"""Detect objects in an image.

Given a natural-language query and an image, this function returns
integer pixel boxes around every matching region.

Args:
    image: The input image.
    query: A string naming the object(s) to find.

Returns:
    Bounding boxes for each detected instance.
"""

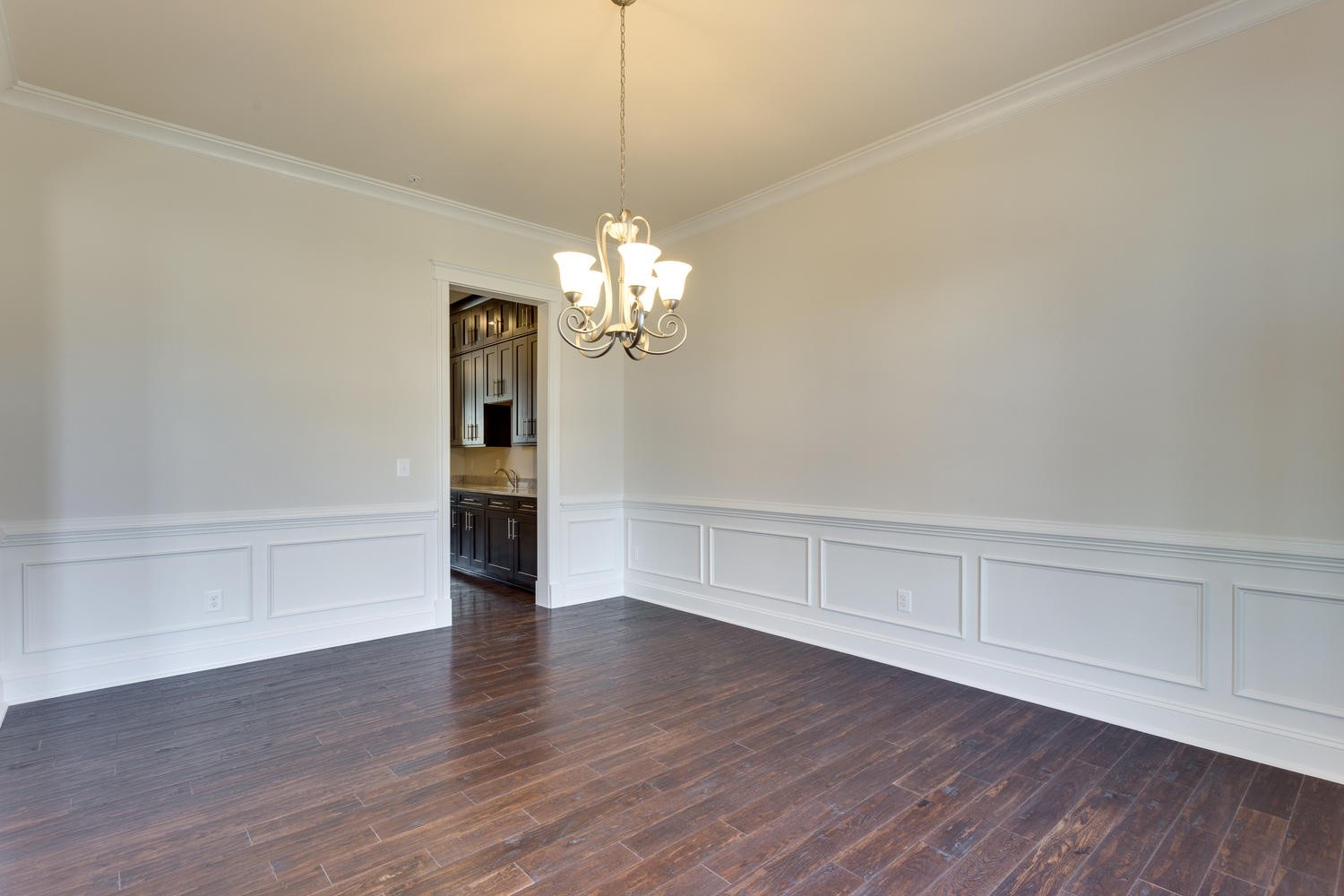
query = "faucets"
[494,468,518,488]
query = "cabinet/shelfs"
[451,491,537,595]
[449,294,538,449]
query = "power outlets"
[495,459,500,469]
[469,459,474,469]
[896,589,911,614]
[206,590,222,614]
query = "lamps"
[552,0,690,361]
